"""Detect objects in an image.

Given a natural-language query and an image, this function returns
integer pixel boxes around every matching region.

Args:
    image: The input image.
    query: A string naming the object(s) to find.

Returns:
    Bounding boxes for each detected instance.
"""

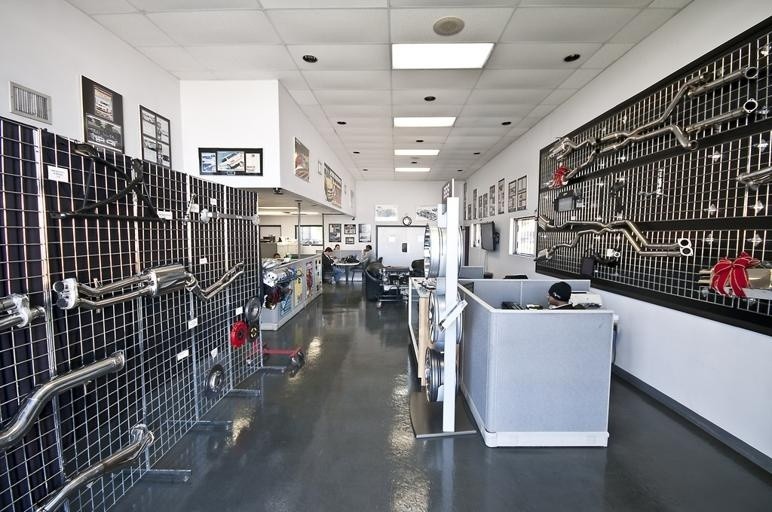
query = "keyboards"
[512,303,527,310]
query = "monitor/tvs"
[480,223,495,251]
[463,282,474,293]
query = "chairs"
[365,269,383,302]
[378,257,383,264]
[410,259,424,277]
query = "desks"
[385,267,409,285]
[384,284,409,302]
[334,262,361,285]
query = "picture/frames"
[329,224,341,242]
[359,224,371,242]
[345,237,354,244]
[344,224,356,234]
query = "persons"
[322,247,345,283]
[333,244,340,250]
[358,245,376,268]
[547,281,574,310]
[274,253,280,259]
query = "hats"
[548,282,571,302]
